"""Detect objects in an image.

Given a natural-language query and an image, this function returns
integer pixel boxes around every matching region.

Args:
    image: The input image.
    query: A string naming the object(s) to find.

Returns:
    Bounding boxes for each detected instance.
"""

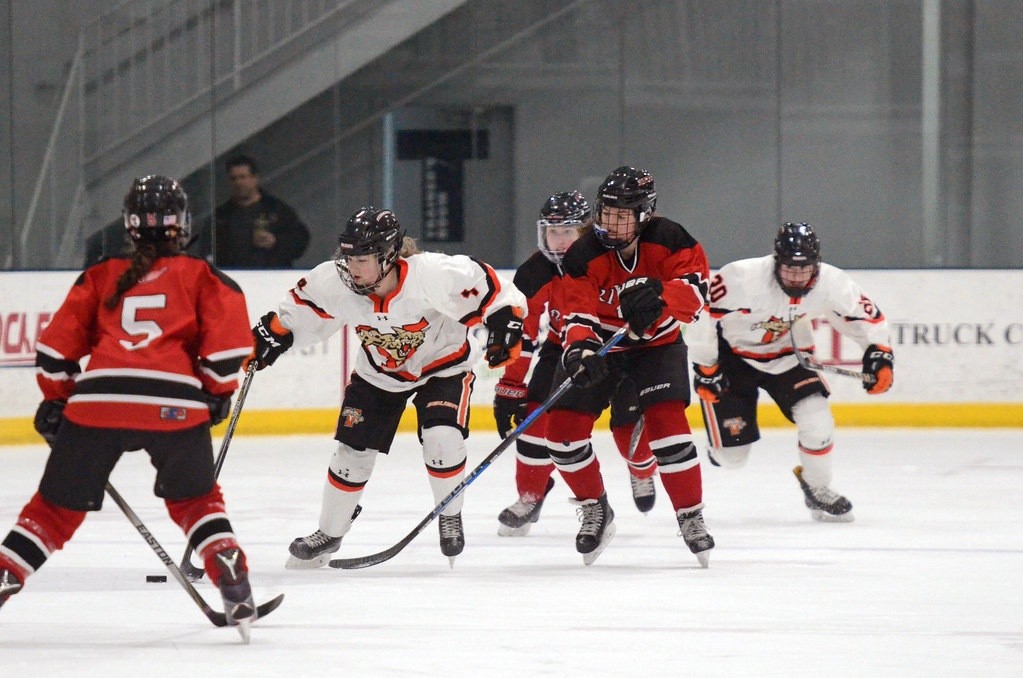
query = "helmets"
[591,166,657,251]
[333,207,403,295]
[123,175,189,248]
[537,189,589,262]
[774,222,821,297]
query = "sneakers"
[676,502,714,567]
[630,473,656,516]
[439,511,465,569]
[569,473,616,565]
[793,465,854,522]
[215,548,258,644]
[284,505,362,569]
[497,477,555,535]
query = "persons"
[681,221,895,522]
[545,165,716,569]
[494,190,658,537]
[200,156,310,270]
[242,206,528,570]
[0,174,256,646]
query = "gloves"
[241,311,294,374]
[861,344,895,394]
[203,388,230,426]
[483,306,525,368]
[617,277,665,338]
[34,399,67,447]
[692,361,729,402]
[563,340,610,387]
[492,378,529,440]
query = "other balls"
[146,574,168,583]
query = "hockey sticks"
[179,358,258,581]
[328,293,665,570]
[45,412,285,629]
[787,311,876,383]
[628,413,643,460]
